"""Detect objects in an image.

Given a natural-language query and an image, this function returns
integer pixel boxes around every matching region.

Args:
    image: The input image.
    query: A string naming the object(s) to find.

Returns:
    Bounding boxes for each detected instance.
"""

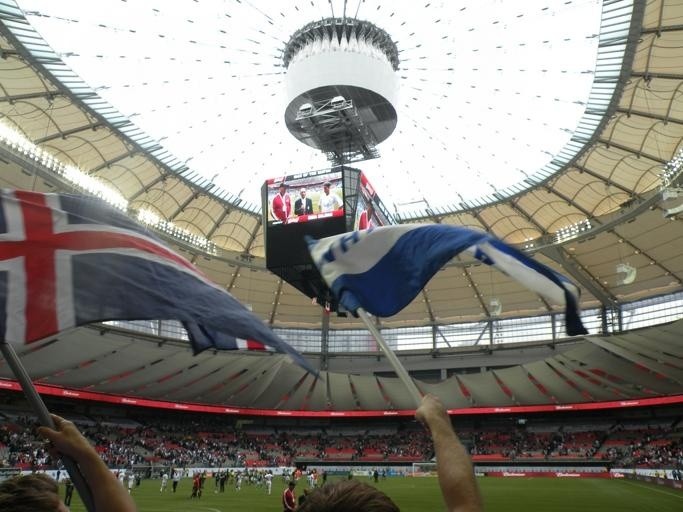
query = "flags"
[0,188,326,383]
[302,220,591,337]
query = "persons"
[1,390,681,512]
[294,188,313,215]
[317,182,344,213]
[269,182,292,224]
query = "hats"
[278,183,289,188]
[323,181,331,186]
